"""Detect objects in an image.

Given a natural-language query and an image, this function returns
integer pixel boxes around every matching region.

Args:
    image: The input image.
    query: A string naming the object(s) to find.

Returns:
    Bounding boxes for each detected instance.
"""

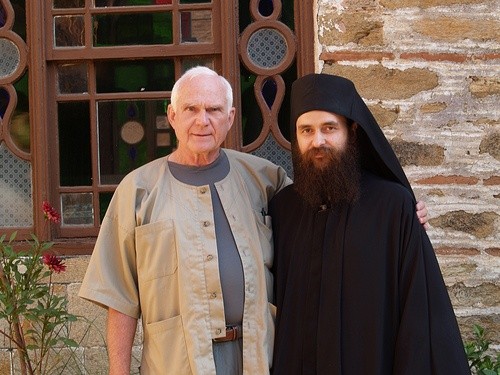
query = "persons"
[77,65,432,375]
[267,72,471,375]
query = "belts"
[211,324,242,342]
